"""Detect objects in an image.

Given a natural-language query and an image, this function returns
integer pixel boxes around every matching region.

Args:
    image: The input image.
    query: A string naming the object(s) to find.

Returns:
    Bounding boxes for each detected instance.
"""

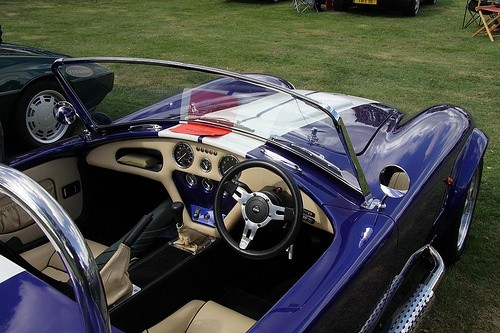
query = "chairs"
[471,0,500,43]
[462,0,499,32]
[140,300,258,332]
[0,231,135,313]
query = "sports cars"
[0,58,487,333]
[0,42,114,151]
